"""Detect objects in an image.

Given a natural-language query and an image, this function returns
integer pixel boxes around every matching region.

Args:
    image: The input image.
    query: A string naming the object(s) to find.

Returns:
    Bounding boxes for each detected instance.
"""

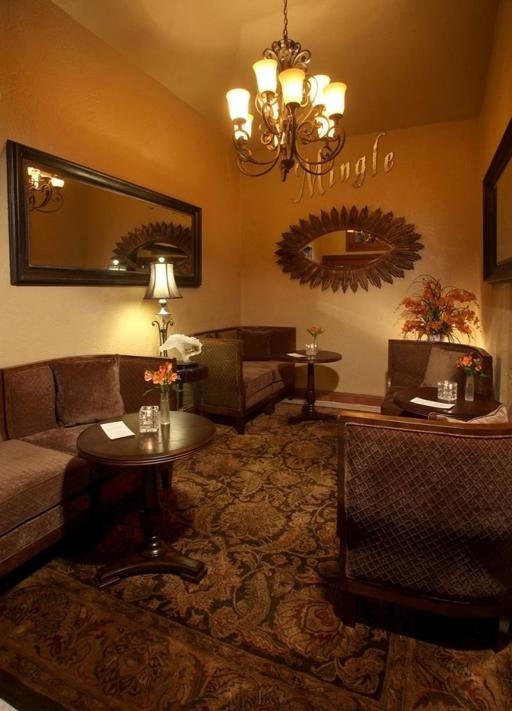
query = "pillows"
[238,328,274,361]
[428,404,509,426]
[49,354,125,427]
[418,346,468,389]
[3,365,58,440]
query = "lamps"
[142,257,183,357]
[225,0,349,183]
[27,166,65,214]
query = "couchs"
[336,407,511,626]
[185,326,297,434]
[1,354,178,578]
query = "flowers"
[307,325,325,340]
[455,352,488,378]
[141,361,184,397]
[395,273,484,343]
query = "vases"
[428,332,444,344]
[464,375,475,403]
[159,389,171,425]
[305,339,319,356]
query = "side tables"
[174,364,209,416]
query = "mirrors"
[6,138,203,289]
[107,220,194,275]
[273,204,425,294]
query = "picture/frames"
[482,116,512,284]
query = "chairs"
[381,334,493,416]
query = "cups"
[304,342,320,357]
[138,404,161,436]
[437,379,460,400]
